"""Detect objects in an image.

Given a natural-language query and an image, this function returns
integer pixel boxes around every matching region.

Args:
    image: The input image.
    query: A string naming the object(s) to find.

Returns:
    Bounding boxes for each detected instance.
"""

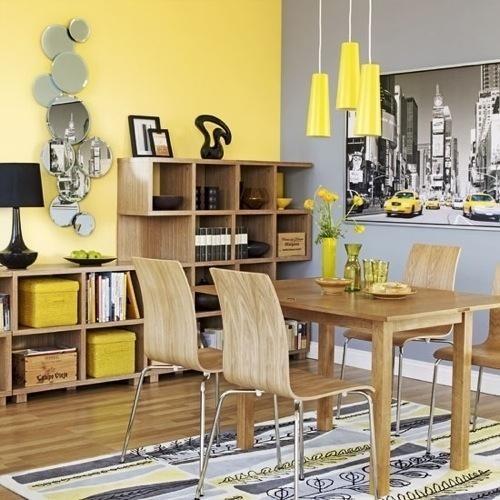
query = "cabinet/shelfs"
[0,259,148,407]
[117,155,313,383]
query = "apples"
[71,249,101,260]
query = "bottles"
[344,243,363,292]
[240,187,269,209]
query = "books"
[12,346,76,357]
[0,293,10,331]
[195,225,250,262]
[87,273,141,324]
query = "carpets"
[0,396,500,500]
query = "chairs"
[193,268,379,500]
[119,256,283,497]
[336,244,462,437]
[426,263,500,455]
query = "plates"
[63,256,118,266]
[314,276,353,288]
[365,289,417,300]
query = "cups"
[371,259,391,286]
[362,259,376,284]
[195,186,202,211]
[204,185,219,210]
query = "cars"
[346,190,370,210]
[422,195,465,210]
[383,190,423,218]
[462,193,500,222]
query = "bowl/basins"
[276,197,293,210]
[152,195,183,210]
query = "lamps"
[0,163,45,269]
[305,0,382,138]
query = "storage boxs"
[19,278,80,328]
[277,232,305,256]
[14,352,77,387]
[86,329,137,378]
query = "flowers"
[304,186,366,245]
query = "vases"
[322,238,336,278]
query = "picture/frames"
[128,115,160,157]
[148,129,173,157]
[344,59,500,233]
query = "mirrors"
[33,18,113,236]
